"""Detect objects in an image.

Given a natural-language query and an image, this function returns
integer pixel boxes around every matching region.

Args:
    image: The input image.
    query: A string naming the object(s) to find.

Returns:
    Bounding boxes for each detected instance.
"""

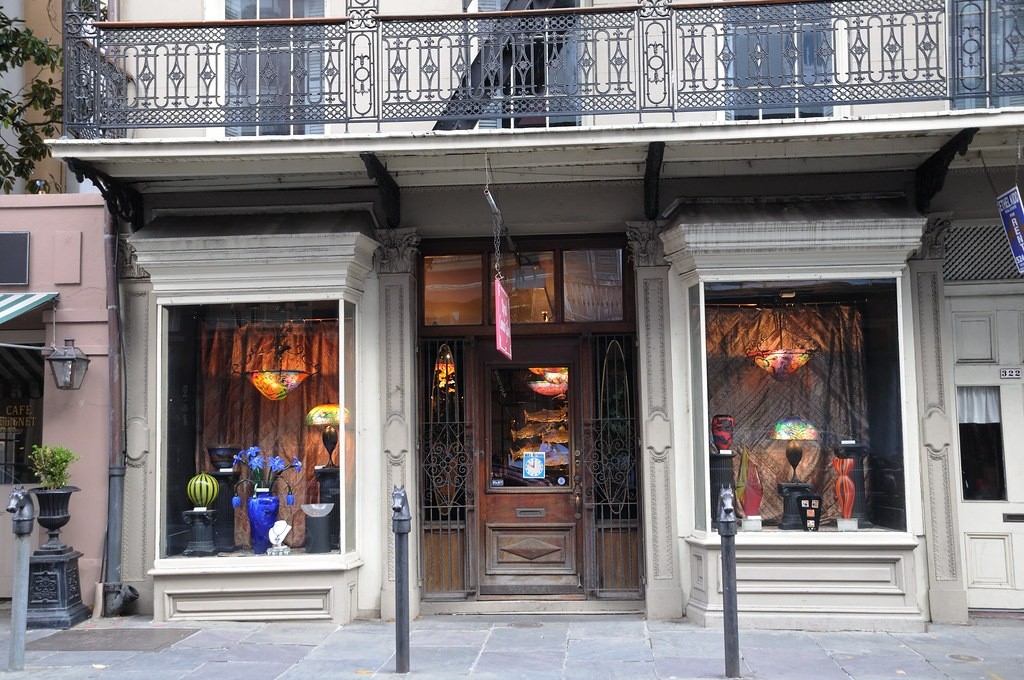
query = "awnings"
[0,292,57,326]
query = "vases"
[247,488,280,554]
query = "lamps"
[527,367,568,400]
[745,305,817,382]
[770,413,820,482]
[303,403,351,468]
[231,320,321,401]
[46,339,90,390]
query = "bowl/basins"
[300,503,335,517]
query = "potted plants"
[27,444,82,548]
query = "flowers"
[231,446,302,509]
